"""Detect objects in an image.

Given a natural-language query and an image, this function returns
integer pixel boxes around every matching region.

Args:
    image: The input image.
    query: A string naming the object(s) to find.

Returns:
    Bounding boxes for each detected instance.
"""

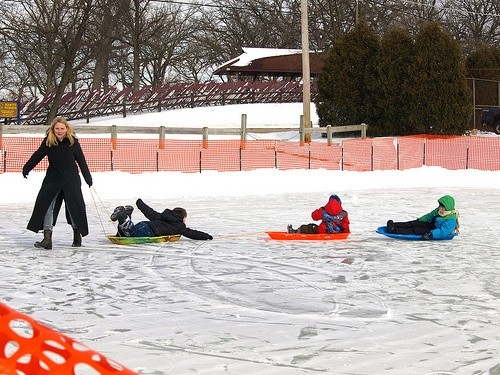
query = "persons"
[386,194,460,240]
[287,194,351,234]
[23,117,93,249]
[110,198,213,240]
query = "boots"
[72,227,82,246]
[35,231,53,249]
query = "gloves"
[208,235,213,240]
[421,233,432,241]
[23,170,29,179]
[86,181,93,187]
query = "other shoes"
[287,224,295,233]
[111,206,126,221]
[387,219,395,233]
[122,206,134,217]
